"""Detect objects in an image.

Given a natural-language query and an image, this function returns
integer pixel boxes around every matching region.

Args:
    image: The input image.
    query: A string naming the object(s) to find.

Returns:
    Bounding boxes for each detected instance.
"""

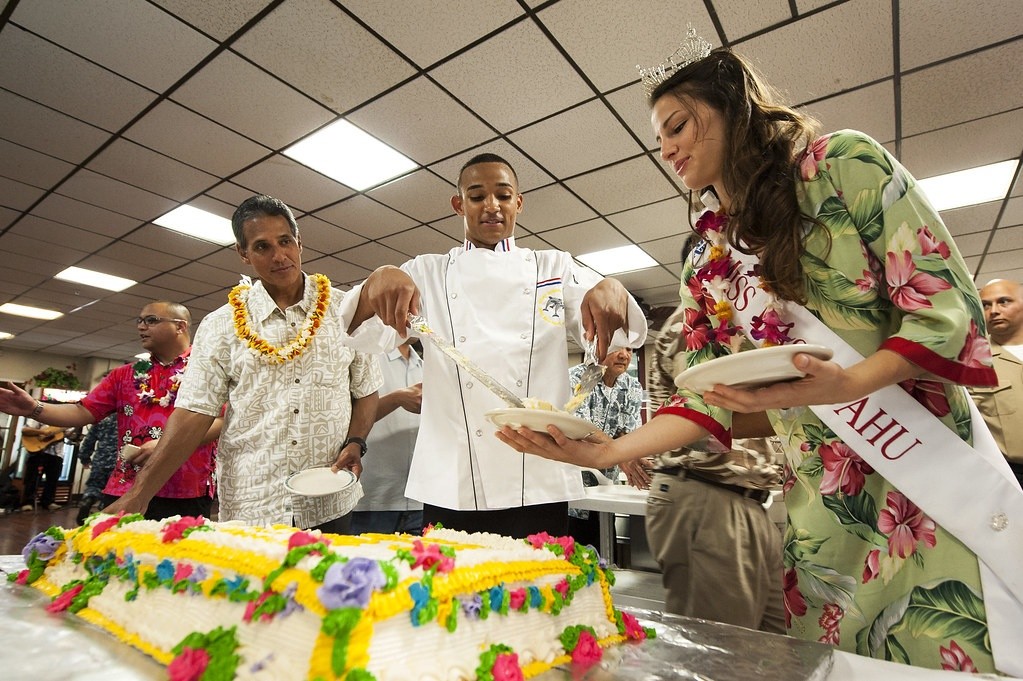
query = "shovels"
[567,325,608,414]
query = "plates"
[285,468,357,497]
[674,344,833,396]
[483,407,596,441]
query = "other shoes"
[22,505,32,510]
[47,503,62,510]
[76,499,92,525]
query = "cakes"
[520,398,555,413]
[8,511,657,681]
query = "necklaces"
[228,273,331,368]
[131,357,190,407]
[694,208,796,347]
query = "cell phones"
[121,444,141,461]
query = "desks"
[568,482,787,566]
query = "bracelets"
[341,437,368,458]
[31,400,44,420]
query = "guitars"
[22,426,77,453]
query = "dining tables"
[0,555,1000,681]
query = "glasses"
[137,315,187,324]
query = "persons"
[0,300,225,525]
[338,152,648,544]
[102,195,386,534]
[349,314,424,536]
[490,23,1022,681]
[568,317,652,517]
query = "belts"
[653,465,770,503]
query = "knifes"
[406,313,525,408]
[560,327,607,413]
